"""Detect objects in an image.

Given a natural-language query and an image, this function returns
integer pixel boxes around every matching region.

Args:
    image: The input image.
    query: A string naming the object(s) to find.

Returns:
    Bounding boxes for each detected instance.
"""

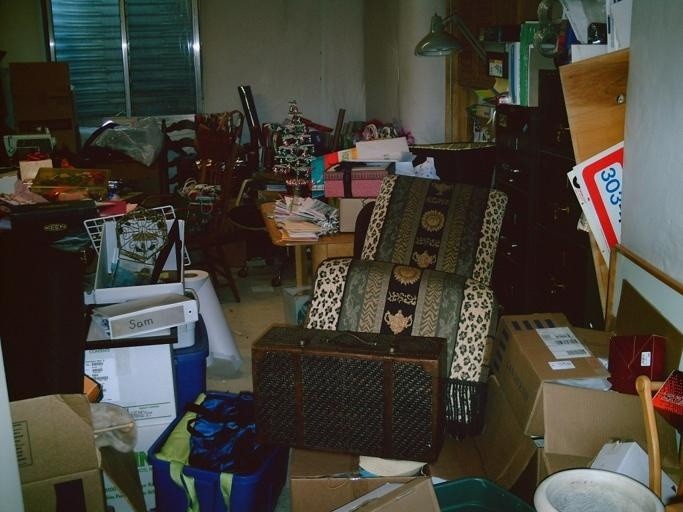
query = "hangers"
[415,16,465,57]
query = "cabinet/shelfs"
[526,1,625,330]
[0,199,99,400]
[447,0,530,316]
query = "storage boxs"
[10,394,104,512]
[173,315,209,413]
[87,218,186,306]
[571,329,622,370]
[146,386,287,512]
[492,314,613,438]
[333,481,406,511]
[432,476,536,511]
[542,381,681,512]
[650,369,681,437]
[289,448,442,511]
[608,335,667,398]
[473,375,540,492]
[355,476,440,511]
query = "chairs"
[143,117,242,304]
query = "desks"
[265,196,354,296]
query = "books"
[506,21,555,107]
[268,195,337,242]
